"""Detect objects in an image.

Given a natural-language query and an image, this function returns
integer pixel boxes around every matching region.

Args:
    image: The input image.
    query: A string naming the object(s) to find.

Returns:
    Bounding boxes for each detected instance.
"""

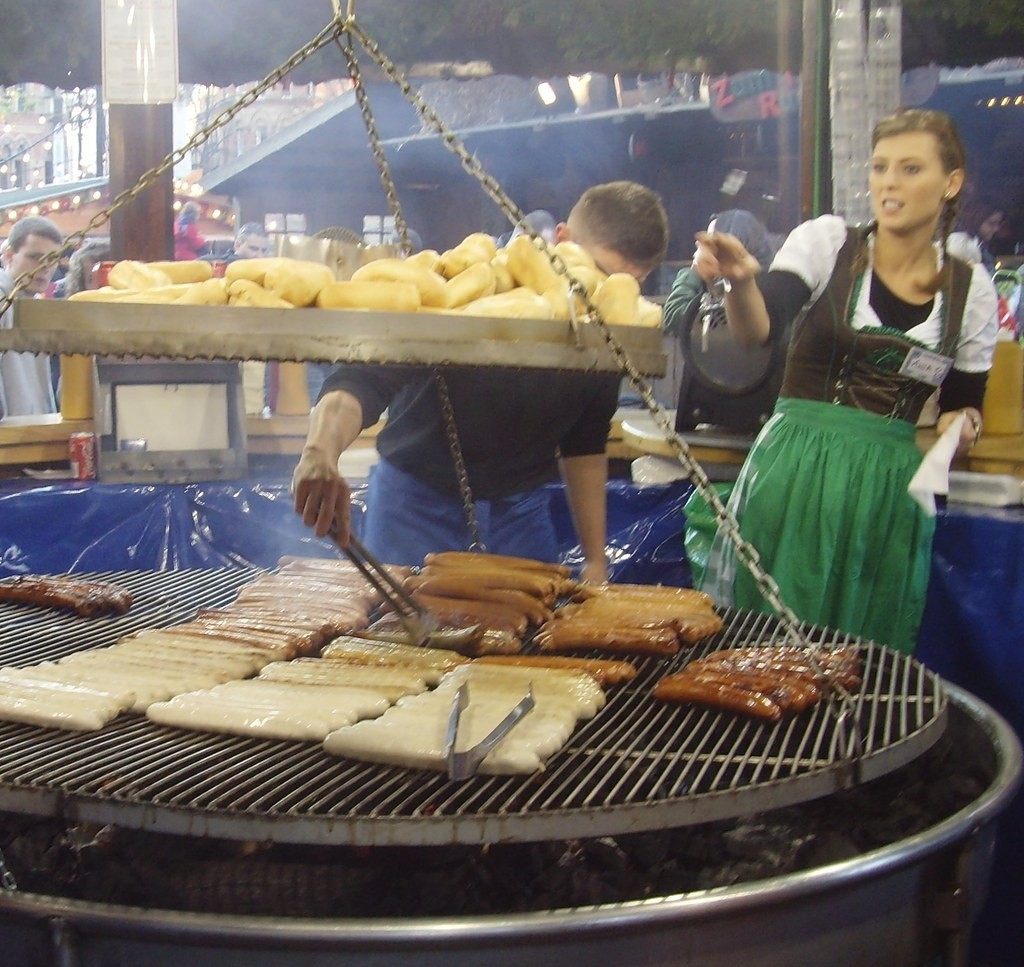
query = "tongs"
[445,677,535,781]
[288,482,440,645]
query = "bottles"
[980,327,1022,437]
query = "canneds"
[69,432,97,480]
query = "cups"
[569,72,710,115]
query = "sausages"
[0,552,861,777]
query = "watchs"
[957,408,981,453]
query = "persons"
[0,215,115,420]
[944,195,1005,297]
[194,220,268,264]
[696,109,1001,664]
[291,181,670,639]
[660,207,783,437]
[174,201,205,264]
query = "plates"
[23,464,71,479]
